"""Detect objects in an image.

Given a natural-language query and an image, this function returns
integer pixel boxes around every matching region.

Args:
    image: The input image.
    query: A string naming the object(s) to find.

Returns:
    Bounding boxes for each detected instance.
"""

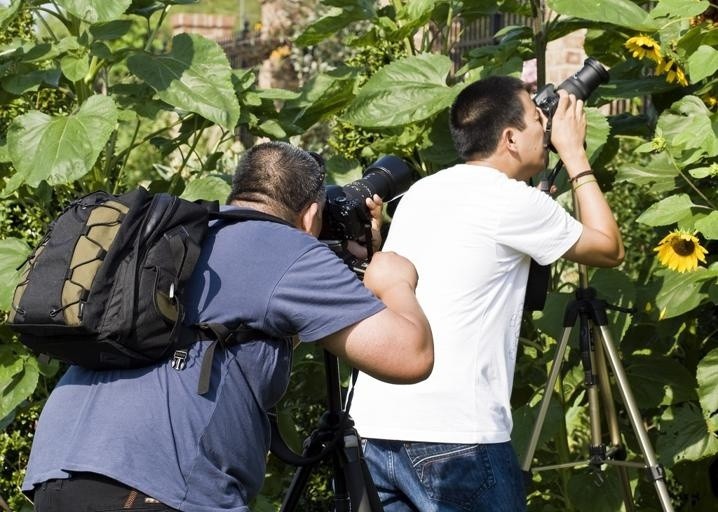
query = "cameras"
[532,56,609,151]
[322,154,411,243]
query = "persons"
[343,75,626,512]
[18,142,434,512]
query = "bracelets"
[567,169,595,184]
[572,179,599,192]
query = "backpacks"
[4,184,297,374]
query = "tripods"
[518,152,676,512]
[274,349,384,512]
